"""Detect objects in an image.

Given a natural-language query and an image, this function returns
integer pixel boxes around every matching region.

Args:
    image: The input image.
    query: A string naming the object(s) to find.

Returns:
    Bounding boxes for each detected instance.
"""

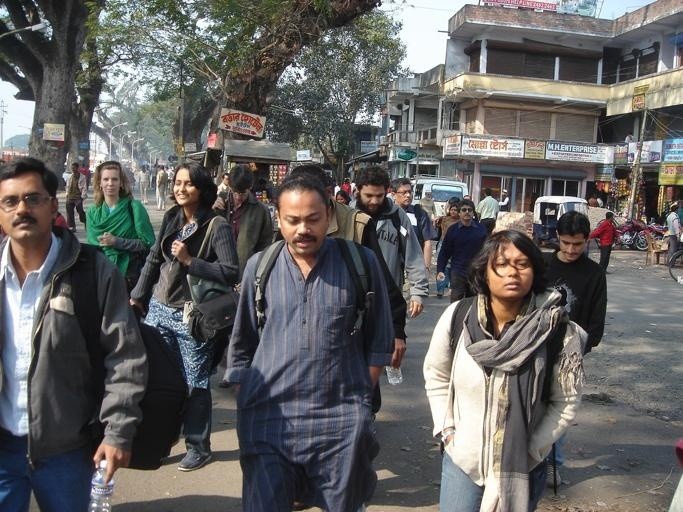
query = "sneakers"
[216,366,232,389]
[175,448,214,473]
[436,289,444,297]
[68,227,77,233]
[545,457,565,487]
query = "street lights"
[130,137,145,164]
[149,150,159,169]
[131,137,145,160]
[154,151,163,164]
[1,22,47,40]
[148,145,158,150]
[146,143,163,170]
[0,18,45,36]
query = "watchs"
[425,267,431,273]
[440,430,456,442]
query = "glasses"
[394,190,414,194]
[0,192,55,212]
[460,208,474,212]
[231,187,248,194]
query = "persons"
[84,160,157,291]
[0,157,146,512]
[463,195,479,222]
[447,197,461,205]
[255,178,274,201]
[498,189,511,212]
[223,176,397,512]
[130,162,238,471]
[213,165,279,310]
[290,165,411,412]
[678,201,683,228]
[424,230,584,511]
[669,196,682,209]
[351,165,430,317]
[333,175,357,208]
[667,203,683,267]
[65,163,92,231]
[432,206,460,296]
[418,190,438,221]
[437,199,488,306]
[126,164,175,213]
[535,210,608,486]
[217,173,231,192]
[475,188,499,235]
[588,212,615,274]
[388,177,432,313]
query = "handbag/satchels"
[123,198,151,294]
[189,218,240,347]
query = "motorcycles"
[594,212,652,252]
[650,225,668,251]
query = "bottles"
[385,366,404,386]
[89,459,115,511]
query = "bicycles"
[668,248,683,283]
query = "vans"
[410,178,468,227]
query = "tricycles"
[532,195,590,256]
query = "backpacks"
[65,244,185,472]
